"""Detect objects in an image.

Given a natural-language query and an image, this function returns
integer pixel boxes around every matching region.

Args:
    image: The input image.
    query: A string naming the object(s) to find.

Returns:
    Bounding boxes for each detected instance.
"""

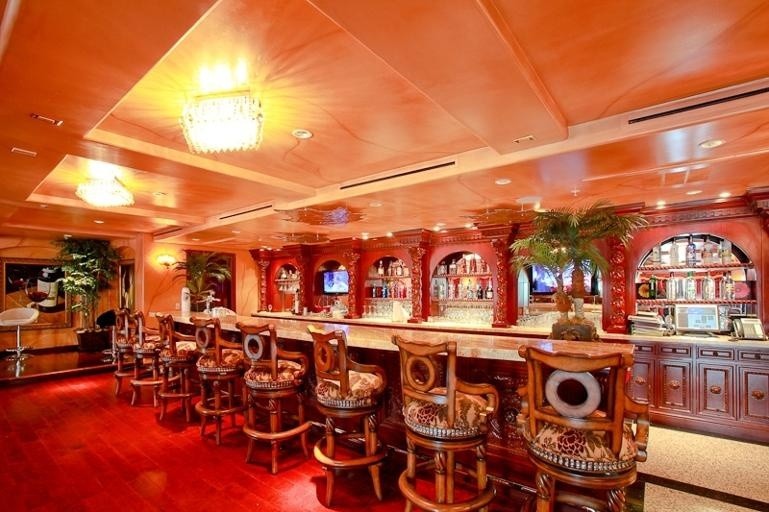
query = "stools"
[309,329,397,508]
[390,331,501,512]
[114,309,151,396]
[154,312,201,424]
[234,320,311,475]
[126,310,178,406]
[518,342,652,511]
[188,316,248,445]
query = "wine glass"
[7,269,51,323]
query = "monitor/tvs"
[321,269,349,295]
[530,258,592,293]
[675,303,720,337]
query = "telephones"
[732,318,766,341]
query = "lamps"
[76,91,262,210]
[157,254,177,268]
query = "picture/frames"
[0,257,74,331]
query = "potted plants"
[49,237,123,352]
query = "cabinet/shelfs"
[247,184,769,341]
[625,338,769,447]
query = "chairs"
[0,307,40,379]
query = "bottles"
[686,234,697,265]
[37,265,61,313]
[666,272,677,300]
[722,234,731,263]
[652,240,661,264]
[670,237,679,266]
[372,259,412,298]
[684,271,697,301]
[701,272,715,301]
[719,270,735,300]
[703,234,714,264]
[433,253,493,300]
[648,271,658,299]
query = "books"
[626,308,664,336]
